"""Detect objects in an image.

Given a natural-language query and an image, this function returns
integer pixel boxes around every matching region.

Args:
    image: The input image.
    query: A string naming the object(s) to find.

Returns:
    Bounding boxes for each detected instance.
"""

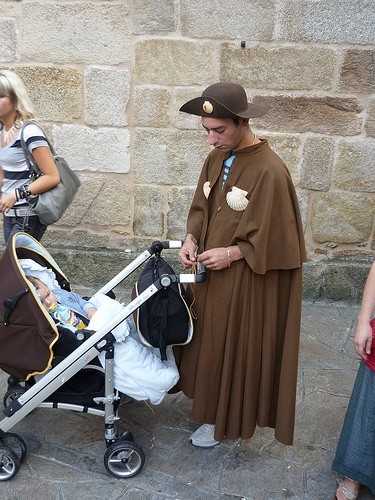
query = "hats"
[179,83,269,117]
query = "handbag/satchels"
[19,122,81,226]
[131,258,193,362]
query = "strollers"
[0,231,207,483]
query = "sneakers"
[189,422,221,447]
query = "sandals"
[335,476,359,500]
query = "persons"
[332,260,375,500]
[24,275,97,331]
[165,83,305,449]
[0,69,60,248]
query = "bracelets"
[15,184,31,201]
[227,246,230,267]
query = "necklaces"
[253,133,257,143]
